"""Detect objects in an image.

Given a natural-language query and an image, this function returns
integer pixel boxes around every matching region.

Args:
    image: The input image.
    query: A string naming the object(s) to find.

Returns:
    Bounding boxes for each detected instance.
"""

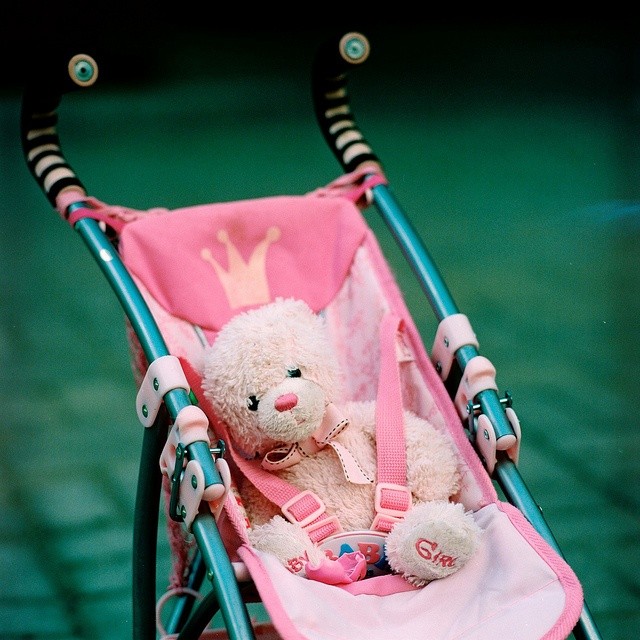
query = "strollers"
[21,27,604,640]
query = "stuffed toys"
[201,298,482,589]
